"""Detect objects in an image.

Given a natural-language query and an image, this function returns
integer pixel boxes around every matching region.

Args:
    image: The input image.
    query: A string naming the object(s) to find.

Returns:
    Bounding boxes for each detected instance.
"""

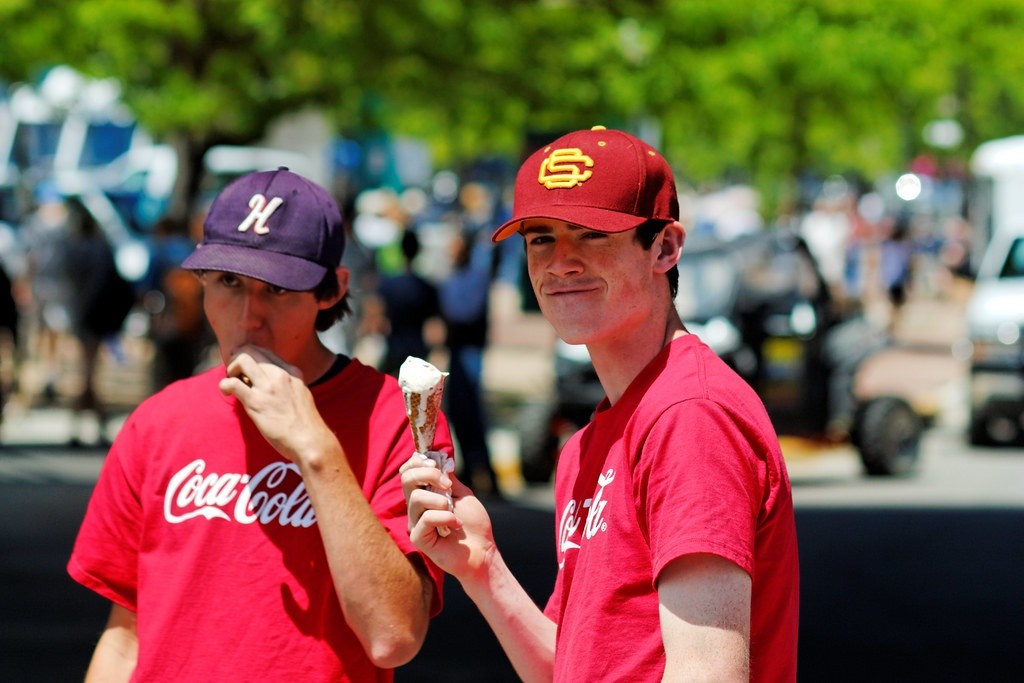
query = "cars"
[516,227,925,486]
[938,215,1024,448]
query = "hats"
[181,167,347,291]
[496,127,680,240]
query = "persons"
[0,195,492,482]
[400,124,799,683]
[66,166,454,683]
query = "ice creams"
[399,355,446,453]
